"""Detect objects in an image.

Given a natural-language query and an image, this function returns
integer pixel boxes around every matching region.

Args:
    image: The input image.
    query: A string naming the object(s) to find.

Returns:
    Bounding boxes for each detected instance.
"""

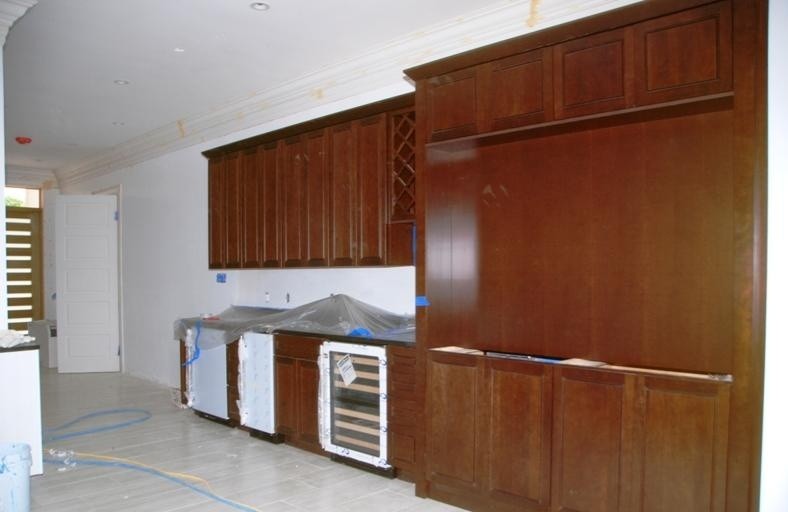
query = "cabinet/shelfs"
[424,45,554,141]
[179,318,417,484]
[555,1,734,121]
[426,346,555,511]
[207,112,388,269]
[552,358,733,510]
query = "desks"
[1,341,45,478]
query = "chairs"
[33,320,57,368]
